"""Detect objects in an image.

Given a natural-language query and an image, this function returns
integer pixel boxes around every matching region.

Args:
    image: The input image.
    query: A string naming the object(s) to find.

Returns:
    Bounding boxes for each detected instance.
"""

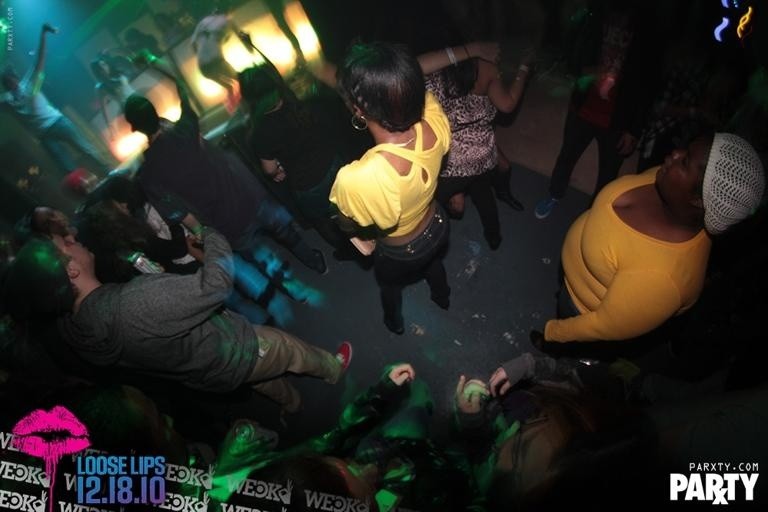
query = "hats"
[701,132,764,236]
[2,239,73,315]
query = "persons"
[318,32,456,332]
[2,340,767,512]
[5,220,352,408]
[0,2,768,321]
[526,128,766,364]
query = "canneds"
[234,422,279,449]
[131,255,164,275]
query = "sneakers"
[308,248,330,275]
[535,197,556,219]
[334,341,353,385]
[529,330,561,360]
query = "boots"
[496,169,523,210]
